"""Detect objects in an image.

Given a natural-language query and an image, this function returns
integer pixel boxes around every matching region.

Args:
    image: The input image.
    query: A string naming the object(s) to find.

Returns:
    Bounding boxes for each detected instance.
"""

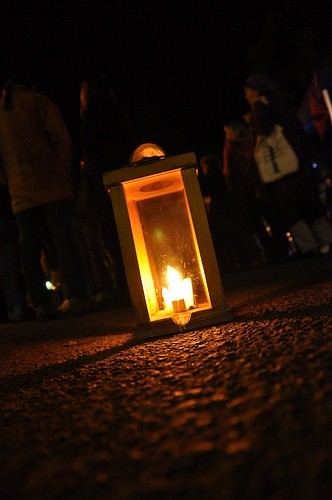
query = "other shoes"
[1,280,125,322]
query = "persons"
[1,58,100,321]
[223,72,332,259]
